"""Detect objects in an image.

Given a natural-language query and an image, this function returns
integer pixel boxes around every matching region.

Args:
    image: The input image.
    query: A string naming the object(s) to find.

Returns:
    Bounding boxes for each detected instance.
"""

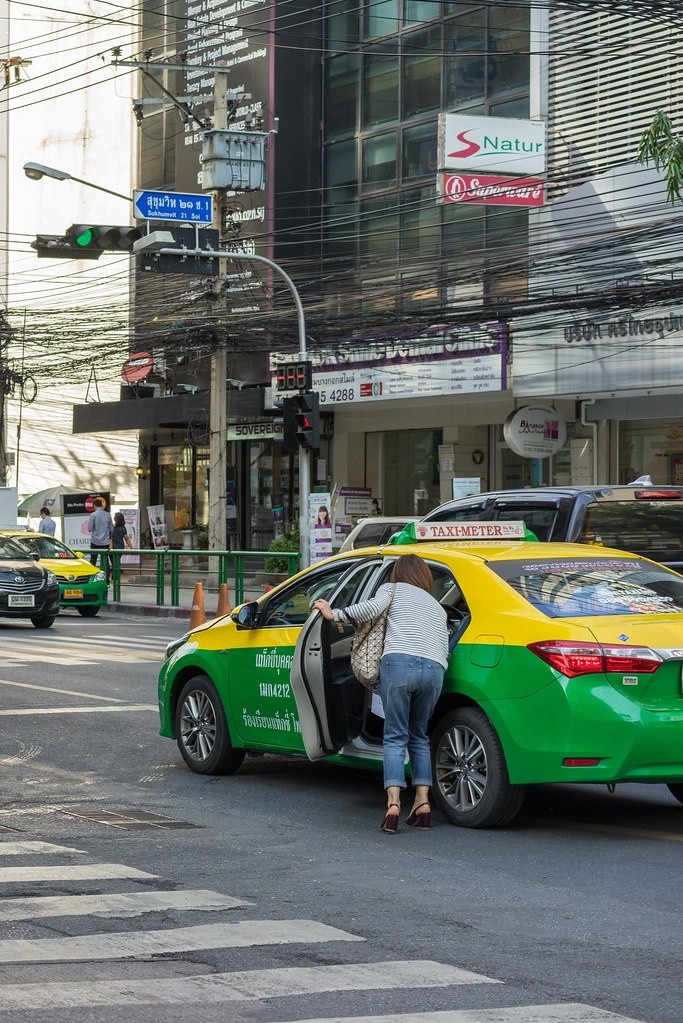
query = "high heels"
[380,804,399,832]
[405,802,432,829]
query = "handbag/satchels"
[109,539,113,549]
[350,582,397,692]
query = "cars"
[156,519,683,829]
[337,514,428,555]
[0,534,60,628]
[0,528,109,618]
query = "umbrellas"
[18,484,93,514]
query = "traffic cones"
[215,583,231,617]
[189,581,207,632]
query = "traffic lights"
[273,397,297,444]
[291,391,320,449]
[67,223,142,250]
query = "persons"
[318,505,330,527]
[88,499,114,588]
[110,512,132,580]
[313,554,450,833]
[38,508,56,536]
[371,499,381,515]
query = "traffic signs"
[132,190,215,221]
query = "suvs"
[419,482,683,575]
